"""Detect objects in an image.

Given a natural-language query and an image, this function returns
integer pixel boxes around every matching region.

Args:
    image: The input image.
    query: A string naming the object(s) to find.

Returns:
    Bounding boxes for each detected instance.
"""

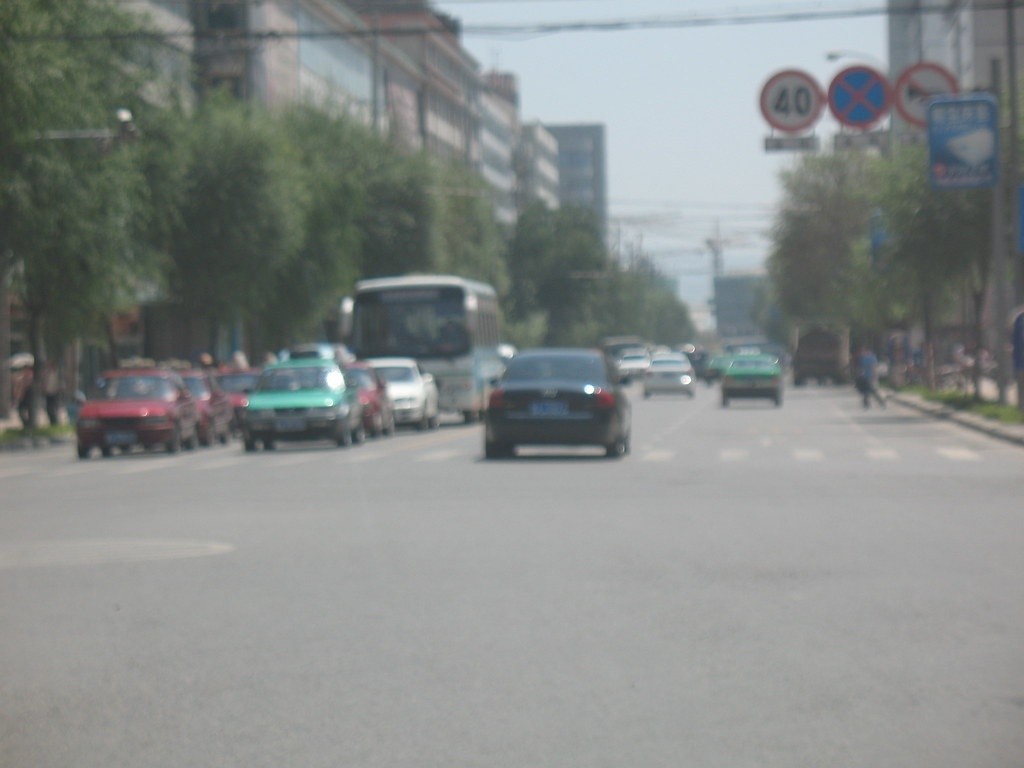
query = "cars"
[75,368,199,459]
[642,351,695,398]
[617,348,650,384]
[682,338,771,385]
[171,344,343,447]
[482,346,630,459]
[235,356,365,452]
[335,360,398,438]
[719,353,784,406]
[367,353,442,432]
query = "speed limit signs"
[760,69,826,135]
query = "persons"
[10,352,286,432]
[851,342,989,409]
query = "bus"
[339,274,506,425]
